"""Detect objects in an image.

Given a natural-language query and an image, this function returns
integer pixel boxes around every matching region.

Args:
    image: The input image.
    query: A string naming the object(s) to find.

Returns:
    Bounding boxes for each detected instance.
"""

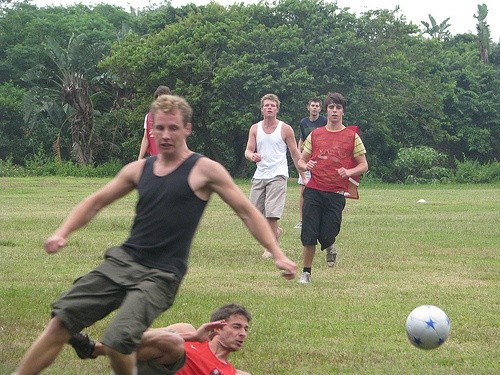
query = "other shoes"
[294,219,302,230]
[277,227,283,235]
[262,248,272,258]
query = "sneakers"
[300,272,311,284]
[326,244,337,267]
[70,332,98,361]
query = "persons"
[296,97,328,227]
[69,303,253,375]
[245,94,308,256]
[138,85,174,160]
[10,93,296,375]
[299,93,368,284]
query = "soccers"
[404,304,450,351]
